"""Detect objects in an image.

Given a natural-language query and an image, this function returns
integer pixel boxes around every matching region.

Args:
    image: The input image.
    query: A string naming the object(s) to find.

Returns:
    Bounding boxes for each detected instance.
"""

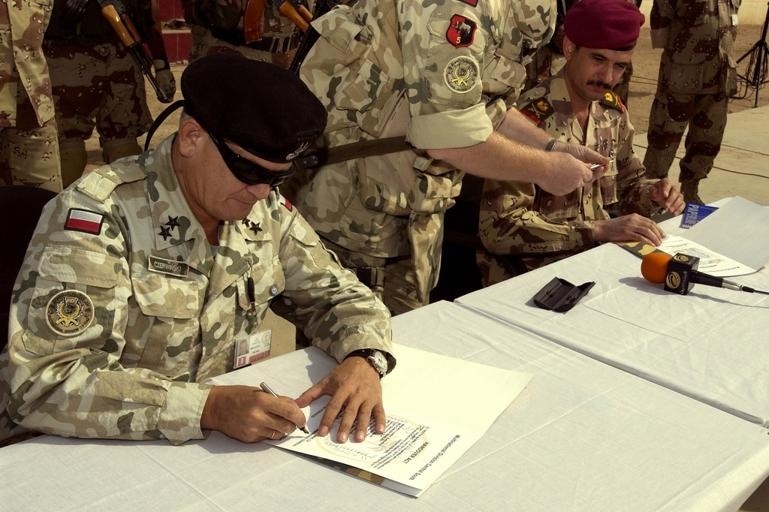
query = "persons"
[473,1,688,293]
[182,1,360,75]
[520,1,645,112]
[40,0,178,192]
[1,52,400,446]
[1,2,63,198]
[292,0,615,352]
[641,1,746,212]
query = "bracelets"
[544,137,569,153]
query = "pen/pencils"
[260,382,309,433]
[589,158,614,170]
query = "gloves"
[153,67,178,105]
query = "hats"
[561,0,646,53]
[177,51,332,165]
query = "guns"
[279,1,313,32]
[102,5,168,100]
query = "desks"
[0,298,769,511]
[453,195,769,427]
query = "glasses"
[203,126,300,189]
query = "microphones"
[639,252,754,294]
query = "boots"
[679,178,706,208]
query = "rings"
[271,429,277,440]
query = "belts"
[208,24,304,55]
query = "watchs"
[346,346,389,381]
